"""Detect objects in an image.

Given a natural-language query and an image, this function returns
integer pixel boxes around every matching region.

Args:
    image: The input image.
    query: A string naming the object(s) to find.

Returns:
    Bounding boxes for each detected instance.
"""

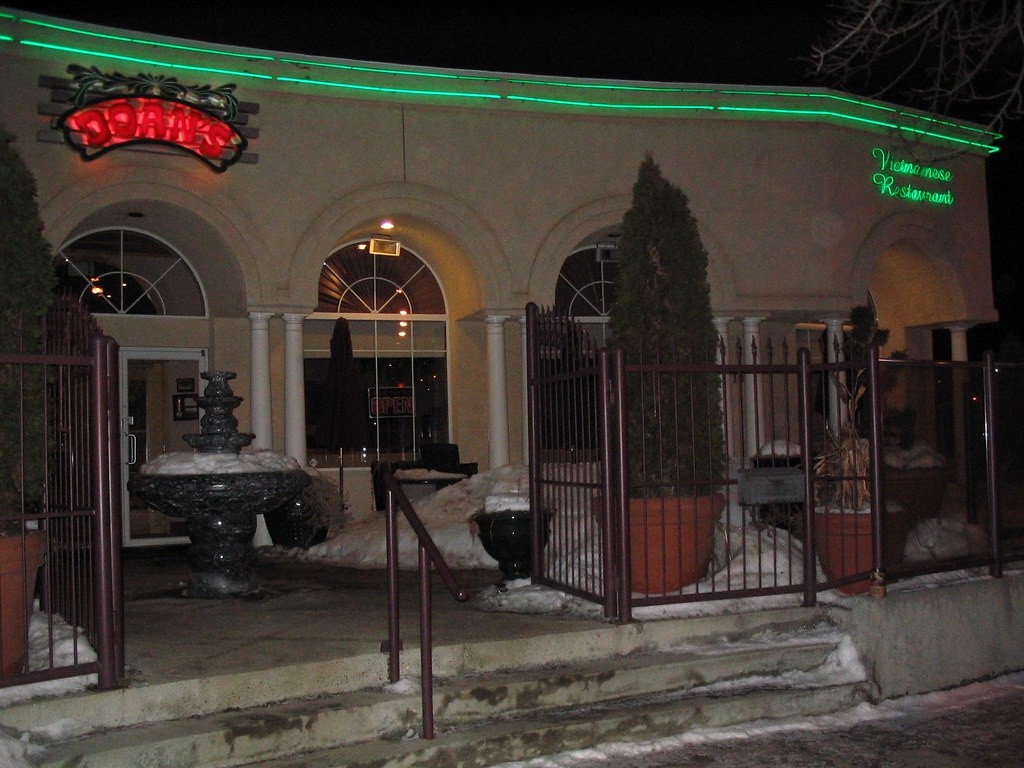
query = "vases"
[891,467,946,524]
[263,479,331,551]
[813,507,910,594]
[473,511,555,583]
[752,453,816,532]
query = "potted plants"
[0,120,60,679]
[592,146,726,593]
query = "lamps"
[368,234,401,257]
[177,378,195,393]
